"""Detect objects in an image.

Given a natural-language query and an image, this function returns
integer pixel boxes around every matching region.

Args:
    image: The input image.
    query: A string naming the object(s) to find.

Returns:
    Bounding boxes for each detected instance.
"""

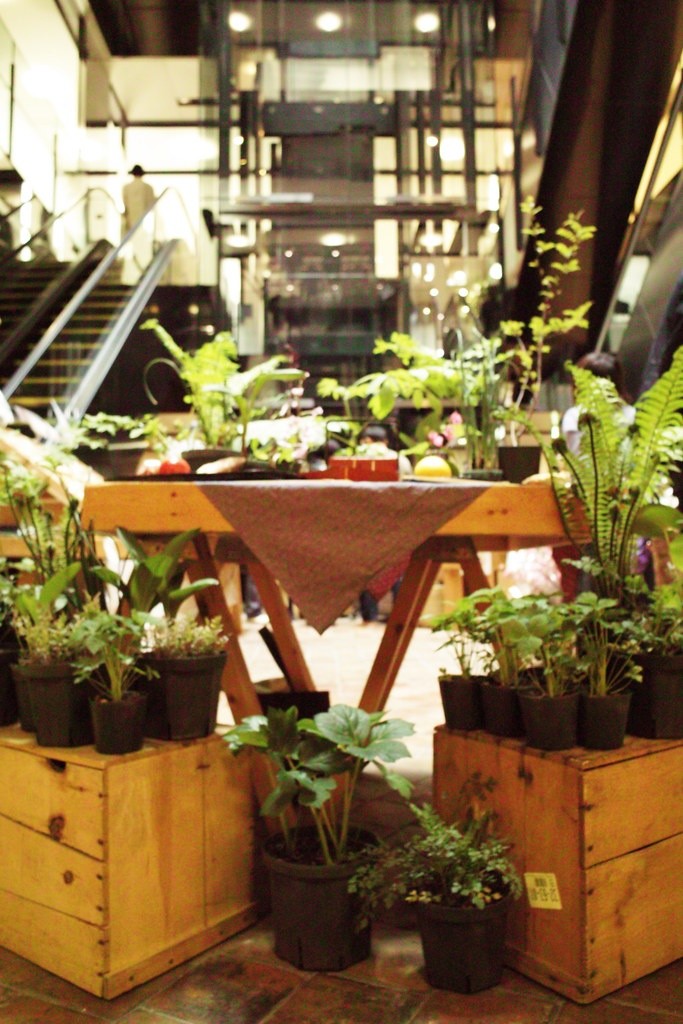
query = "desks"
[68,474,586,837]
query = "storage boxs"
[431,724,682,1006]
[1,716,259,1000]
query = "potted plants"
[347,798,522,994]
[1,192,682,758]
[220,703,419,971]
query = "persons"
[122,165,156,233]
[562,352,635,687]
[241,427,414,622]
